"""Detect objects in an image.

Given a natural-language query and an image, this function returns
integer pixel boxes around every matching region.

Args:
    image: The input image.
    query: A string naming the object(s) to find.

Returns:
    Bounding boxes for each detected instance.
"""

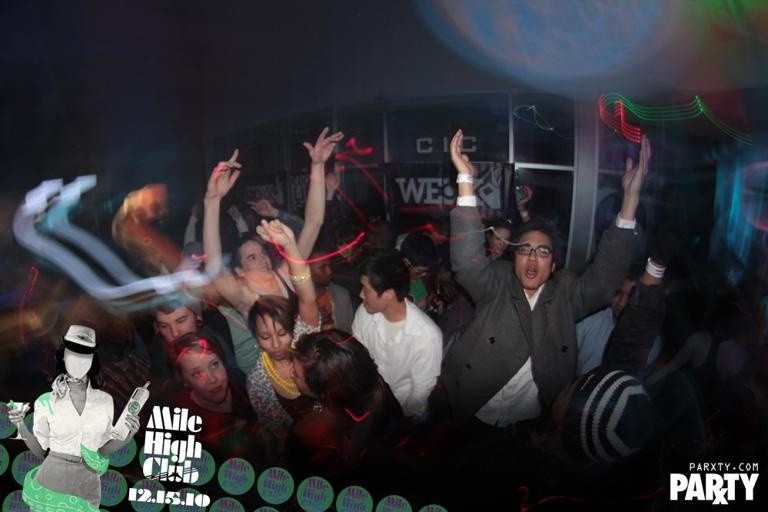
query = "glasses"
[515,244,553,257]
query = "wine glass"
[7,402,28,441]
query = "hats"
[568,365,653,470]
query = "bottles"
[112,379,153,441]
